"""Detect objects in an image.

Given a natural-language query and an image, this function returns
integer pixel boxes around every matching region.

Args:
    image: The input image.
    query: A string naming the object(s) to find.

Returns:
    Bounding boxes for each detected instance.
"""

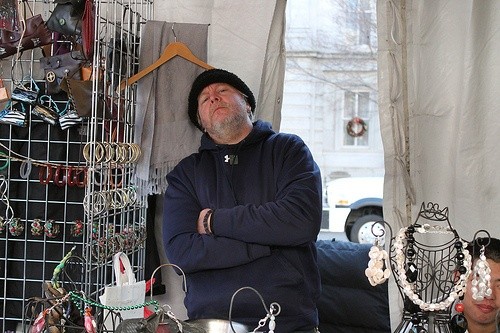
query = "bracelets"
[204,210,212,234]
[0,136,148,260]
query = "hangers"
[117,22,215,91]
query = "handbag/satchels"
[98,252,145,333]
[0,0,129,130]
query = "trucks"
[329,176,389,244]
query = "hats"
[188,68,256,134]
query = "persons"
[163,69,323,333]
[454,238,500,333]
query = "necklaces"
[70,291,160,317]
[394,223,472,333]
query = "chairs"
[316,240,392,333]
